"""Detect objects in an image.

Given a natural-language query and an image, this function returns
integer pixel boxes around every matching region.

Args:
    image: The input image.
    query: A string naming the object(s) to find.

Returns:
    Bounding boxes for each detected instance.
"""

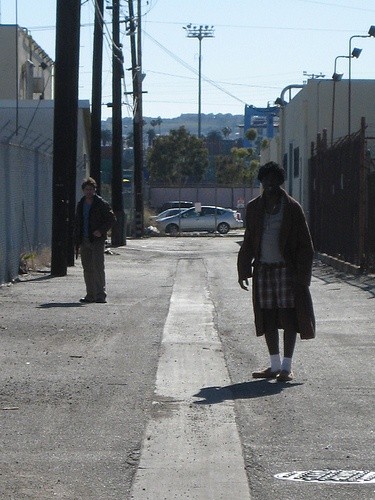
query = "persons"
[237,162,316,382]
[74,177,115,303]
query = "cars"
[157,206,244,237]
[150,208,188,226]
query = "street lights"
[331,48,363,146]
[182,23,217,138]
[348,25,375,133]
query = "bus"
[164,201,193,211]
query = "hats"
[258,162,286,186]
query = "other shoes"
[80,294,96,303]
[252,367,280,378]
[277,370,293,381]
[96,298,105,303]
